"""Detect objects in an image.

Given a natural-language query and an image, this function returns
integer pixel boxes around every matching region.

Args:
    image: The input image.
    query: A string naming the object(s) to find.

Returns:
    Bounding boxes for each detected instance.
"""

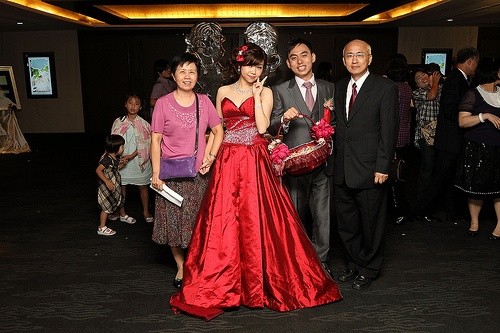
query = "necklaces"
[235,81,255,94]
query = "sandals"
[120,215,136,224]
[97,225,116,236]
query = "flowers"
[311,119,336,140]
[267,138,290,170]
[323,97,336,112]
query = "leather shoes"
[337,269,359,282]
[352,275,375,289]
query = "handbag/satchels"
[158,155,198,180]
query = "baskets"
[268,114,334,178]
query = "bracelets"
[254,104,263,108]
[152,171,161,174]
[211,152,217,157]
[479,113,485,123]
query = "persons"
[150,59,174,116]
[98,94,154,222]
[266,38,335,273]
[169,43,344,321]
[386,47,480,224]
[97,134,136,236]
[333,39,398,290]
[453,61,500,239]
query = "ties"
[303,82,314,111]
[348,82,357,119]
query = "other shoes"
[107,214,119,221]
[145,217,154,222]
[489,233,500,241]
[468,228,479,236]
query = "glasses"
[343,53,370,59]
